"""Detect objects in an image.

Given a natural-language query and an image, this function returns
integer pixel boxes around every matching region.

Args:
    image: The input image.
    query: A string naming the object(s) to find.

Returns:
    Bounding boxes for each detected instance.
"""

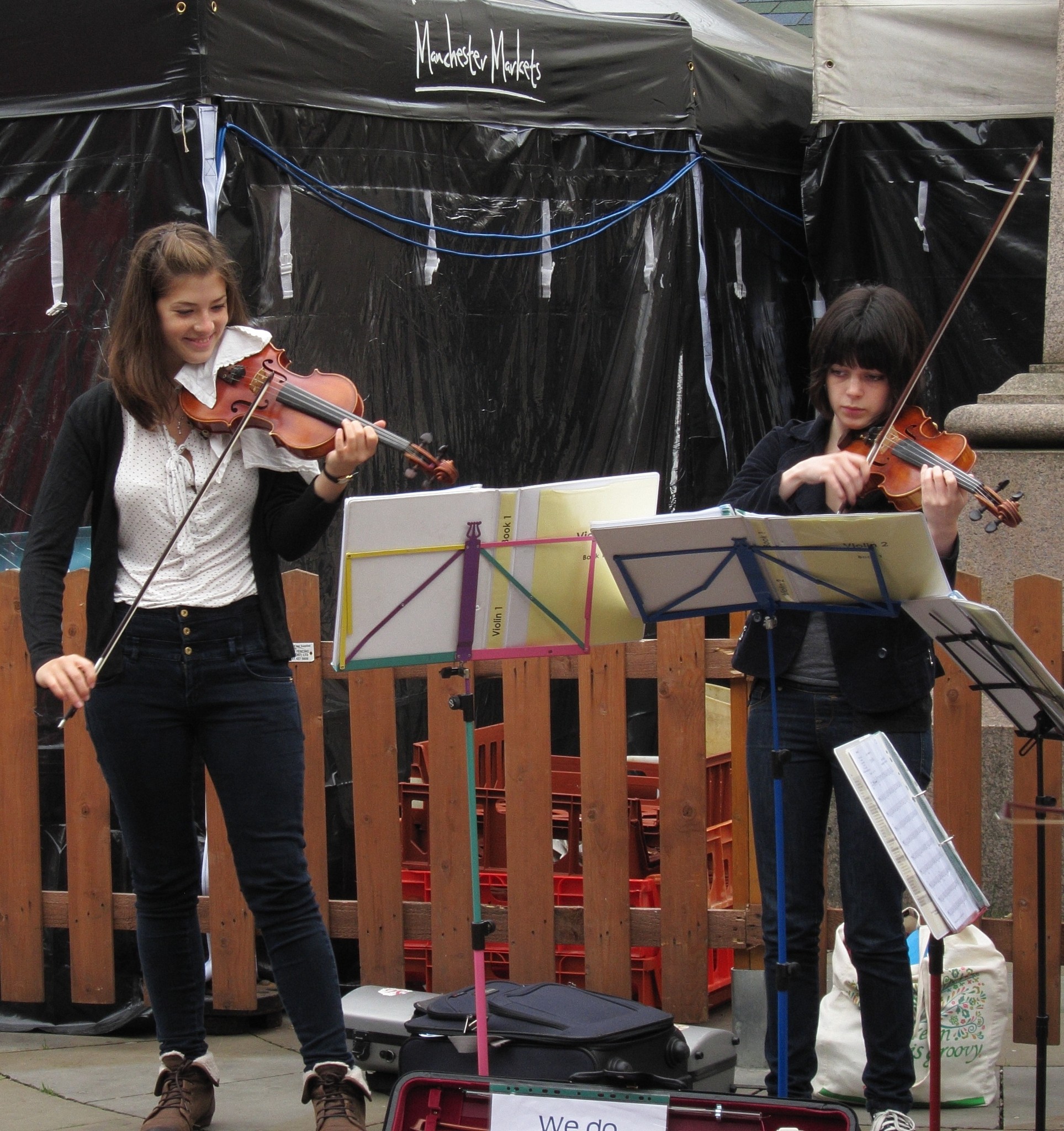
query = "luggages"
[401,980,692,1091]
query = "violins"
[838,404,1026,534]
[179,326,460,492]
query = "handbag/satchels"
[810,908,1005,1110]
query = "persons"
[20,222,385,1131]
[721,284,975,1131]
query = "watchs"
[323,462,361,484]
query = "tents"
[0,0,1064,1035]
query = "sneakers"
[301,1061,375,1131]
[872,1109,917,1131]
[139,1049,220,1130]
